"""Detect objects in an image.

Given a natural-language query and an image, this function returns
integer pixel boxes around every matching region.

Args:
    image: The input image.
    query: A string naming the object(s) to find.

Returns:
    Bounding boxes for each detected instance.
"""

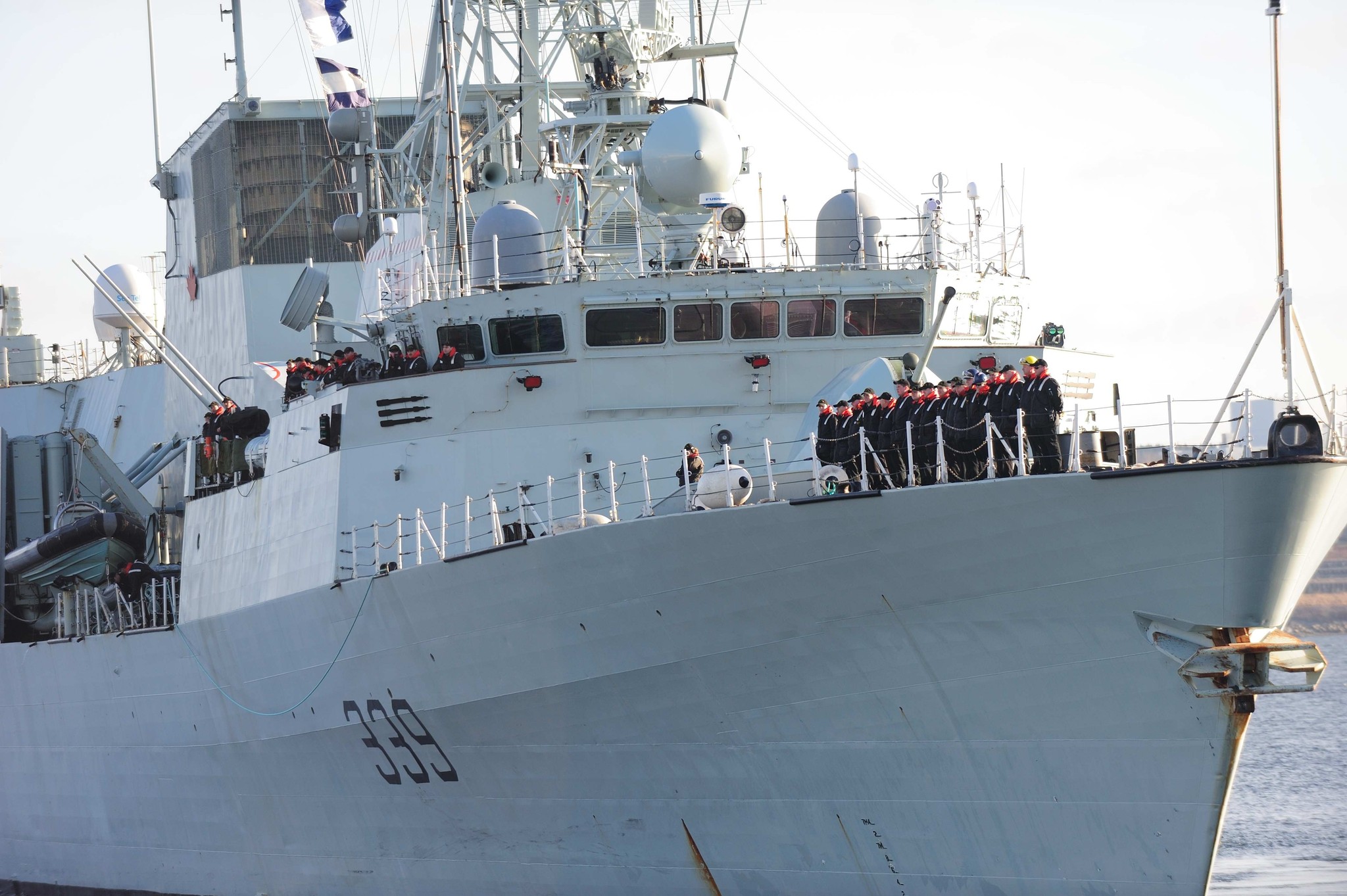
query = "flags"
[298,0,374,118]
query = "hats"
[815,355,1048,408]
[681,443,696,453]
[202,340,454,419]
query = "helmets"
[971,372,988,386]
[1019,356,1038,366]
[962,368,979,379]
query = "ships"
[5,3,1346,896]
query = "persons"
[209,402,234,484]
[110,572,152,628]
[216,396,250,483]
[202,412,225,485]
[284,341,1070,497]
[116,560,174,631]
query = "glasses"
[1033,366,1044,370]
[975,384,985,386]
